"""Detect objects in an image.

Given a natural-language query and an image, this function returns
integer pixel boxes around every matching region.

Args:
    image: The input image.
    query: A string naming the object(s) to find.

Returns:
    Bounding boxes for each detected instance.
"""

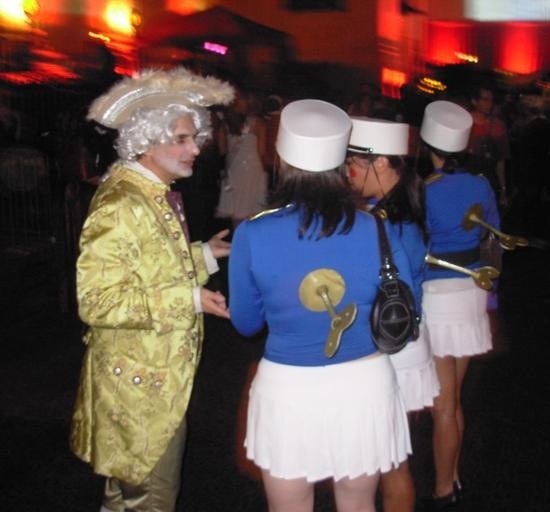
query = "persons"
[337,110,441,511]
[221,95,415,512]
[1,23,550,289]
[413,97,508,511]
[66,67,236,511]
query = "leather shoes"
[419,483,464,512]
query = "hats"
[368,278,421,354]
[347,115,411,158]
[273,98,353,174]
[419,100,474,153]
